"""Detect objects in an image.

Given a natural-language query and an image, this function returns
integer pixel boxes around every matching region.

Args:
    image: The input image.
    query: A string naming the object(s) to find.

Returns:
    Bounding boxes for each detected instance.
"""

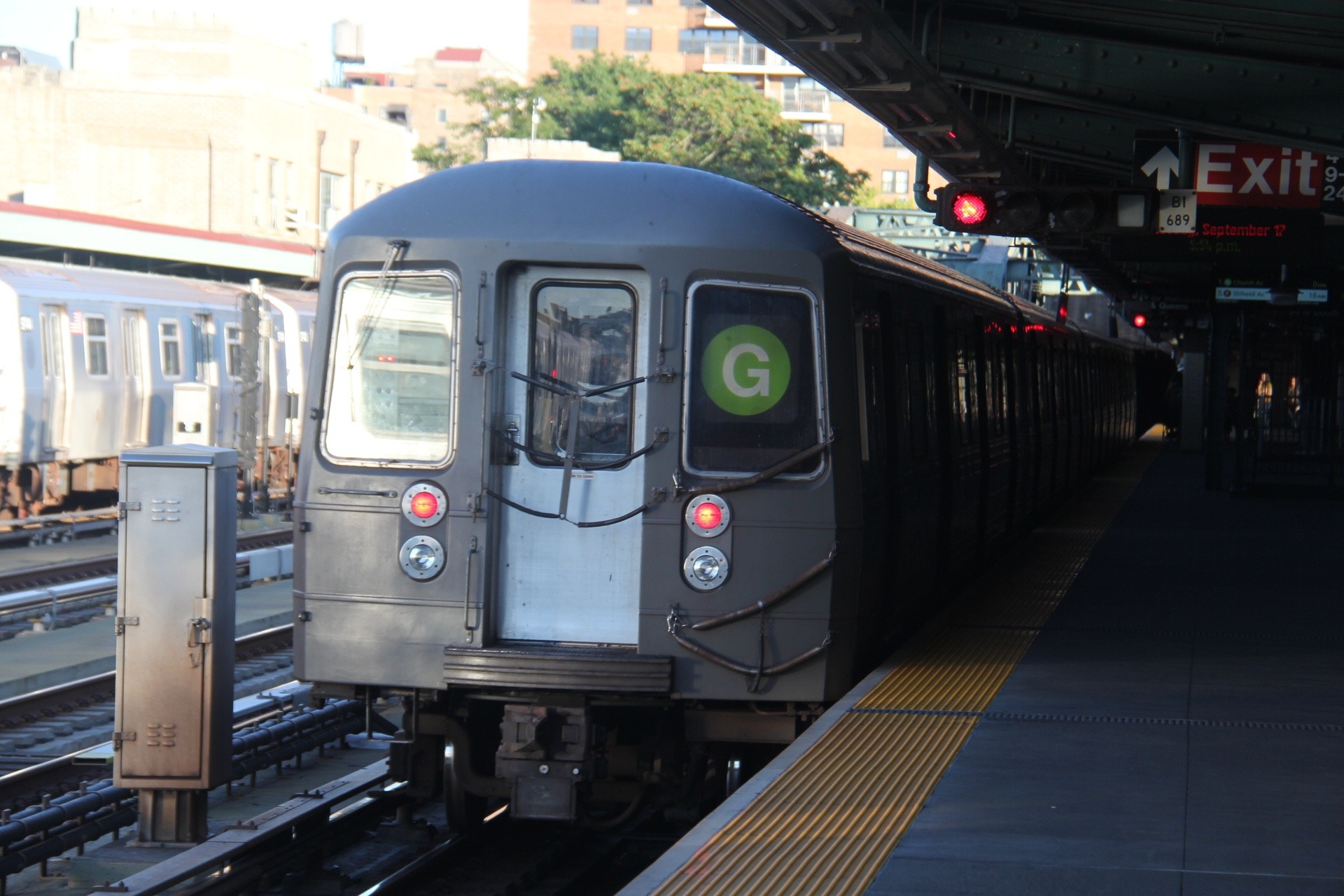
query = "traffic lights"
[1128,312,1149,328]
[932,179,1046,238]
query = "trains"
[0,254,320,521]
[287,157,1184,850]
